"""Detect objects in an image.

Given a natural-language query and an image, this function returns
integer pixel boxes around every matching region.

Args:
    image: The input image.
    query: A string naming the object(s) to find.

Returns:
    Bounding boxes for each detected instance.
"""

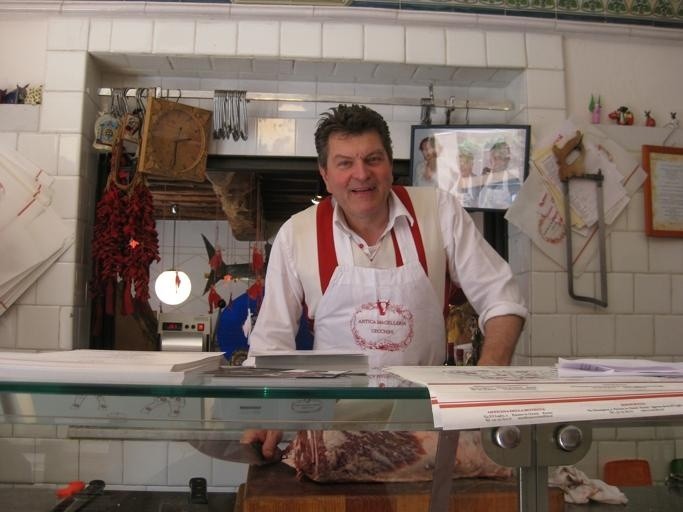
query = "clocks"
[138,95,213,183]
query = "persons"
[447,148,482,209]
[414,136,458,195]
[232,101,530,462]
[477,142,520,210]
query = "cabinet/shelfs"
[1,366,683,512]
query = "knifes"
[187,439,286,466]
[188,477,210,512]
[51,479,105,512]
